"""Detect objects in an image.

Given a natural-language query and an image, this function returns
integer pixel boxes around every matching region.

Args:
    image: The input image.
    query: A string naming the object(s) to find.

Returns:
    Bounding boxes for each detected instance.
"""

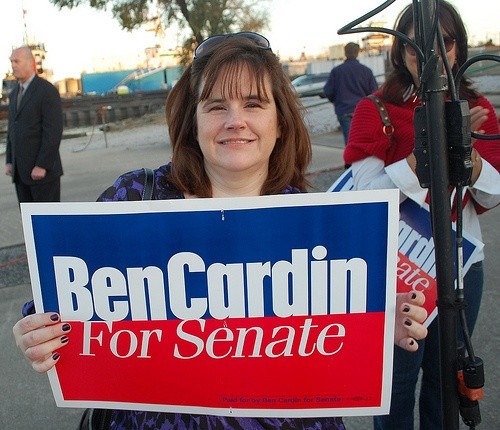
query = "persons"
[13,32,429,430]
[323,42,380,169]
[342,0,500,430]
[3,47,65,214]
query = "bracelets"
[472,148,480,167]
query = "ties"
[17,86,24,110]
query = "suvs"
[289,72,331,99]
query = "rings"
[473,131,478,133]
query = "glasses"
[403,34,457,56]
[194,31,272,60]
